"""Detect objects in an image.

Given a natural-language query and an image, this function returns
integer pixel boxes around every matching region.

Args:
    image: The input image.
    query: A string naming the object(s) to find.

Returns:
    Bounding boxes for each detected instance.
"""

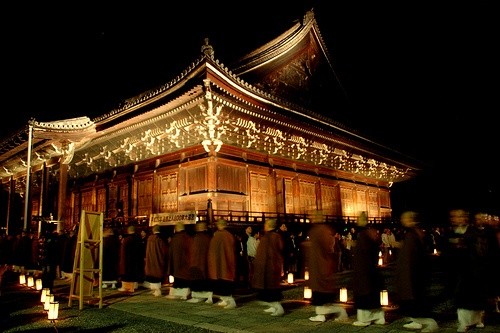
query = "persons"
[0,209,500,333]
[254,220,283,316]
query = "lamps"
[380,290,389,306]
[19,274,59,324]
[304,286,312,300]
[339,287,348,303]
[288,273,294,284]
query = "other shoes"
[352,321,371,326]
[186,298,204,303]
[457,324,468,332]
[402,321,423,329]
[152,291,161,296]
[102,284,108,288]
[270,309,284,316]
[165,294,177,300]
[134,282,138,289]
[421,325,439,333]
[218,300,229,306]
[178,296,188,301]
[264,307,276,312]
[223,302,237,309]
[205,300,213,304]
[374,319,385,324]
[118,287,134,292]
[309,315,326,322]
[476,322,484,327]
[335,316,348,322]
